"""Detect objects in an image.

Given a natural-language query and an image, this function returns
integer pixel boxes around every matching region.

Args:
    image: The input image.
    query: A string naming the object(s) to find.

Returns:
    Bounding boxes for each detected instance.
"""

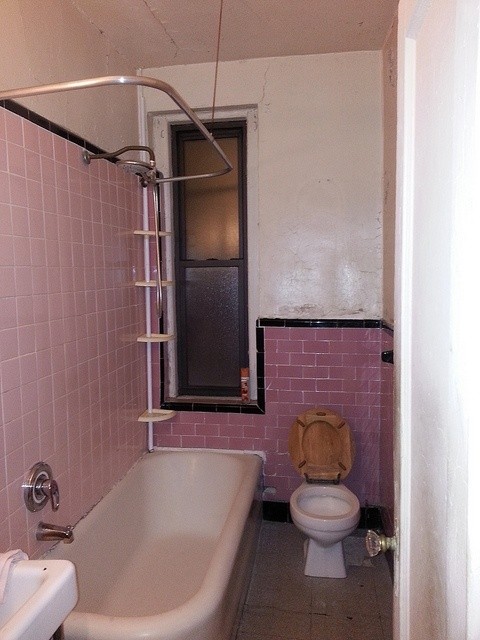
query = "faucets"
[37,523,76,543]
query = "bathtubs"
[44,448,264,639]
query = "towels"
[0,550,29,605]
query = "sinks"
[0,560,79,640]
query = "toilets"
[290,414,361,578]
